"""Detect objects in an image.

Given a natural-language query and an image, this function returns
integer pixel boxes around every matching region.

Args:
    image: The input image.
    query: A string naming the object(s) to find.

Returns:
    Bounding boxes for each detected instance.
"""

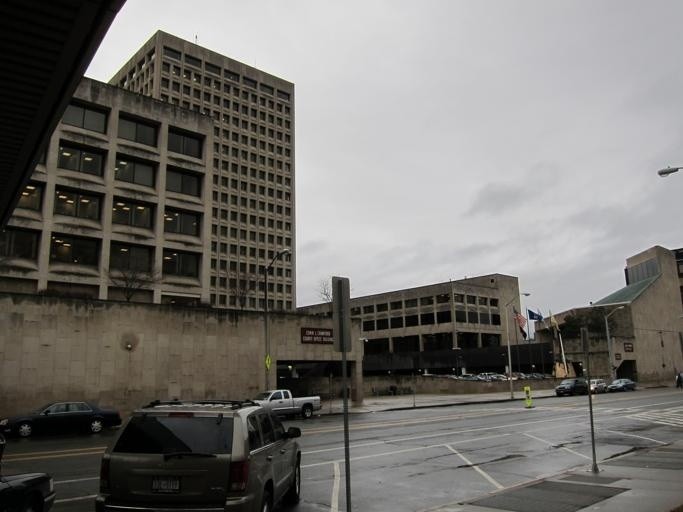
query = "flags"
[528,309,543,323]
[512,311,526,329]
[548,312,562,332]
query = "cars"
[0,400,120,437]
[587,378,607,394]
[609,379,636,393]
[458,372,544,382]
[556,379,588,397]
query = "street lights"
[604,305,624,378]
[504,292,529,397]
[265,249,291,389]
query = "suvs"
[97,398,302,511]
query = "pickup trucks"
[251,389,321,419]
